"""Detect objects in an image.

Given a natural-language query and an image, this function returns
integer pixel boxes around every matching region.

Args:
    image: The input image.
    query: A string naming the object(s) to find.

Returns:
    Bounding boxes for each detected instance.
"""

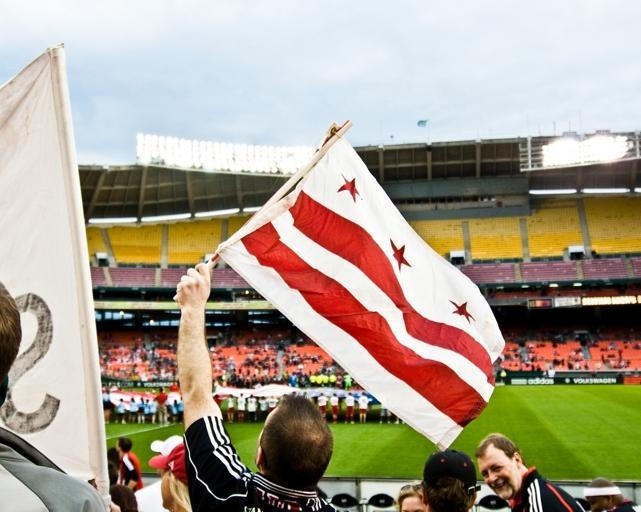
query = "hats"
[149,442,189,485]
[150,434,185,456]
[423,449,482,493]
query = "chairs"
[363,493,399,512]
[473,493,513,512]
[326,491,363,512]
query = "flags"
[0,42,111,512]
[215,124,506,454]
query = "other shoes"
[105,420,262,425]
[323,420,406,426]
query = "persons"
[0,281,641,512]
